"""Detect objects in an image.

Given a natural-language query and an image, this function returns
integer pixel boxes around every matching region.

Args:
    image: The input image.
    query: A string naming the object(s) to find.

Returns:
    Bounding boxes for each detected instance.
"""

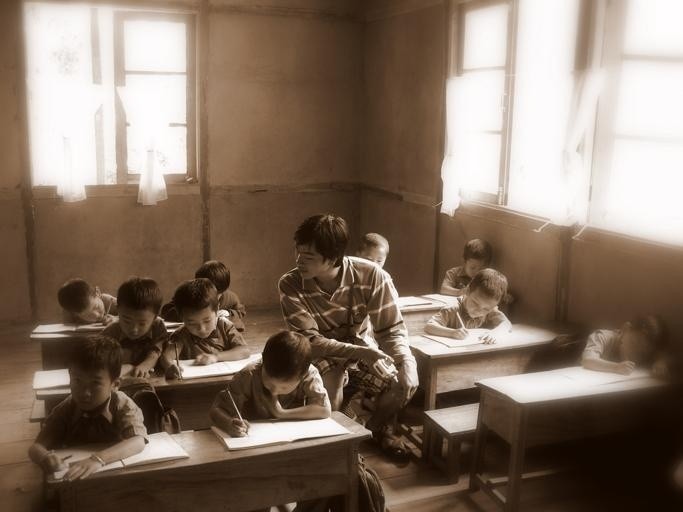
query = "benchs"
[422,403,479,471]
[29,392,47,423]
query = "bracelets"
[39,449,54,464]
[91,454,105,467]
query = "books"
[173,352,263,381]
[210,417,351,451]
[398,295,432,306]
[421,328,490,348]
[32,321,107,334]
[52,431,190,480]
[32,363,135,390]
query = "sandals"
[379,425,411,462]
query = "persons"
[101,277,168,379]
[160,260,246,322]
[159,277,252,380]
[26,335,149,484]
[355,232,390,269]
[579,310,676,379]
[440,237,493,296]
[278,214,421,462]
[423,268,513,345]
[58,277,119,326]
[208,331,333,438]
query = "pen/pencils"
[175,342,182,380]
[106,298,112,316]
[456,312,465,328]
[226,388,248,436]
[62,455,72,460]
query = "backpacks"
[357,454,387,512]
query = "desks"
[399,292,514,335]
[30,313,248,370]
[406,318,593,472]
[467,361,683,510]
[33,355,266,431]
[42,409,373,511]
